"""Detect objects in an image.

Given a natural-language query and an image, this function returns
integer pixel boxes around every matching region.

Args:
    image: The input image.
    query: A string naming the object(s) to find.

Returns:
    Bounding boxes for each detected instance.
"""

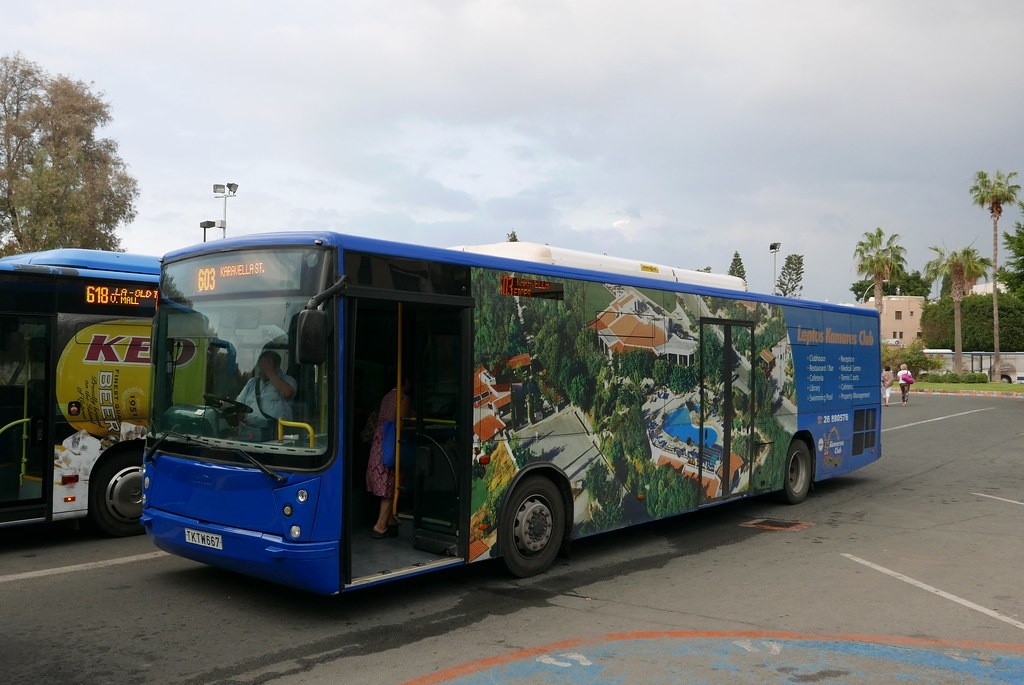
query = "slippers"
[371,527,398,539]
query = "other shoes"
[903,402,906,407]
[885,404,888,407]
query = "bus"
[0,249,286,538]
[138,229,881,596]
[923,349,1024,385]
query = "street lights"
[769,242,782,297]
[213,183,239,238]
[200,221,215,243]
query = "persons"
[219,351,297,429]
[881,366,893,406]
[366,376,411,539]
[536,430,539,443]
[897,364,912,407]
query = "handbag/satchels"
[902,371,916,385]
[382,395,413,469]
